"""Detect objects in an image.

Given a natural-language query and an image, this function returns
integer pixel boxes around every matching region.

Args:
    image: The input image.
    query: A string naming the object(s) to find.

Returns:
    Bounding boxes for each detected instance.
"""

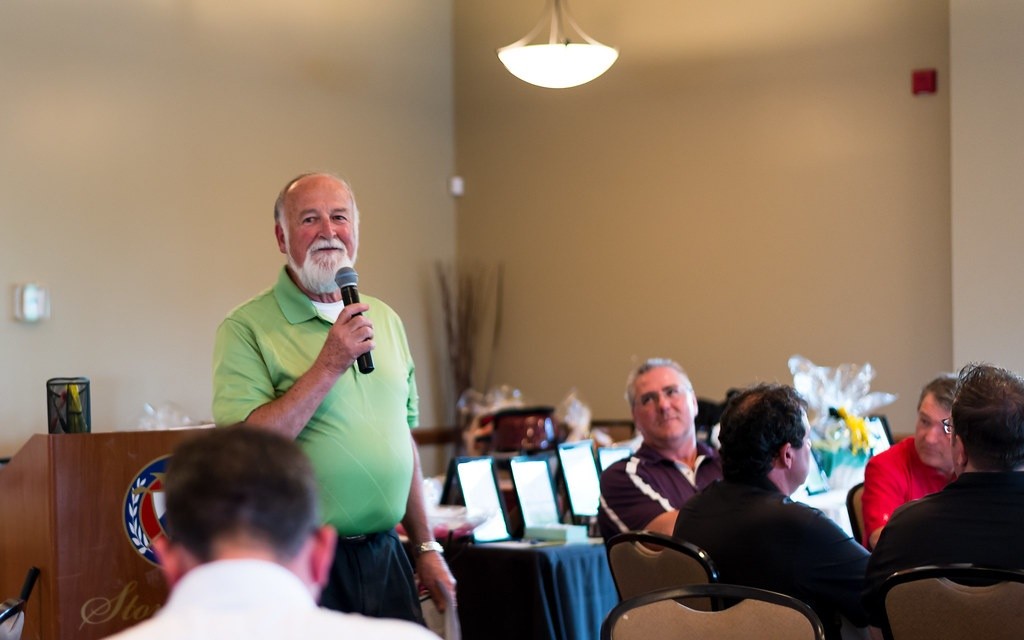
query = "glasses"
[942,417,953,433]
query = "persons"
[672,380,871,640]
[597,359,724,552]
[211,174,457,629]
[843,360,1024,629]
[97,423,444,640]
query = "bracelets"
[410,541,444,555]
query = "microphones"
[334,267,374,374]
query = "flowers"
[496,0,620,89]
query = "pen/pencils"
[49,392,66,433]
[66,384,88,433]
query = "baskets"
[46,378,91,434]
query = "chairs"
[847,481,872,553]
[600,583,826,640]
[874,561,1024,640]
[606,530,720,613]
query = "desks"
[400,469,867,640]
